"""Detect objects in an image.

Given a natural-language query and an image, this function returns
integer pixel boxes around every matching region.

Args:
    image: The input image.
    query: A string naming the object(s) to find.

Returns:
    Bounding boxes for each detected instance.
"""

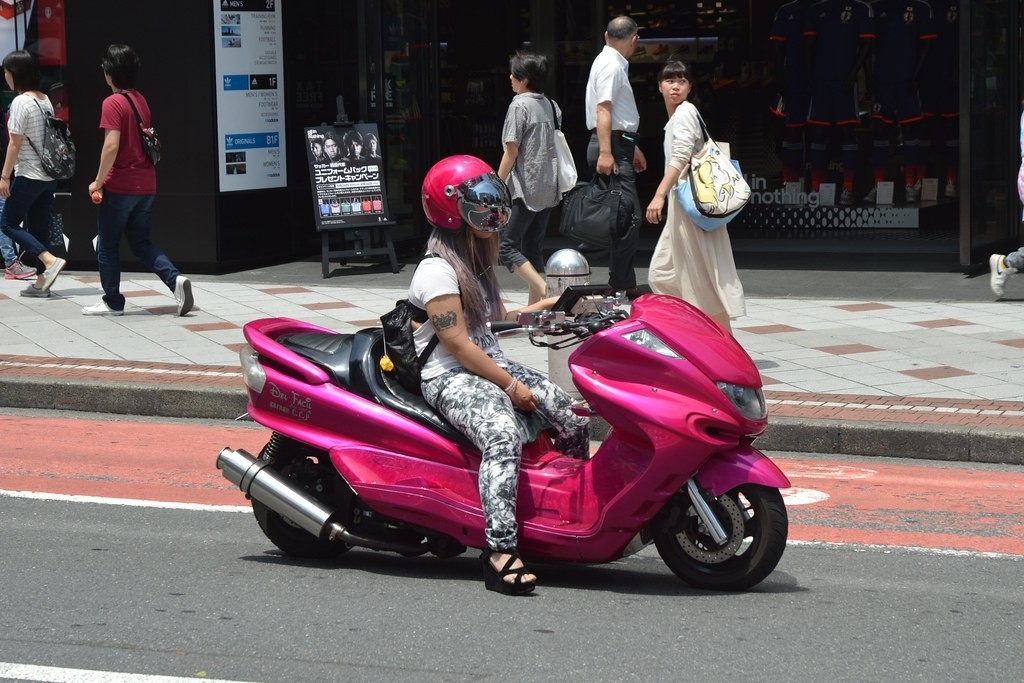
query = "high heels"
[479,547,535,595]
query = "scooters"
[216,287,792,592]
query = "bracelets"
[504,377,517,394]
[1,176,10,179]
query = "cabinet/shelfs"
[439,70,514,157]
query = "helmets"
[422,154,513,234]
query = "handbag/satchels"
[47,198,64,246]
[546,98,578,193]
[558,169,638,249]
[673,105,752,232]
[115,91,161,164]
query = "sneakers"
[5,259,38,279]
[173,275,194,317]
[41,257,67,292]
[20,283,51,298]
[990,254,1018,296]
[81,300,124,316]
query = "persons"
[0,110,37,279]
[320,132,342,163]
[585,16,648,286]
[0,51,67,297]
[408,155,589,595]
[363,133,381,158]
[341,130,365,160]
[989,112,1024,297]
[81,44,194,317]
[645,61,746,335]
[311,138,326,161]
[498,55,561,304]
[769,0,959,207]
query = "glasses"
[632,33,640,42]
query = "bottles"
[92,188,103,205]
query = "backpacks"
[379,253,465,396]
[23,92,77,179]
